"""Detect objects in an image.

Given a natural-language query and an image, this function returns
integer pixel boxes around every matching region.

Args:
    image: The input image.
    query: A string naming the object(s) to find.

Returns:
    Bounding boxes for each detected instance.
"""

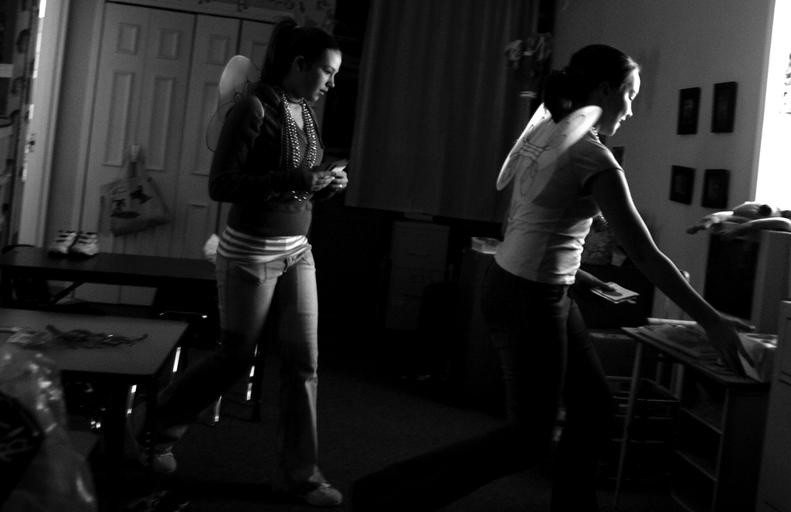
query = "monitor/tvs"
[698,221,791,338]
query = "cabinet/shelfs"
[608,327,767,508]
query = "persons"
[492,44,757,444]
[127,20,349,506]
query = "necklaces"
[280,92,318,202]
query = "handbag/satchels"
[100,175,172,237]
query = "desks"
[0,243,227,478]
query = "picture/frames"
[666,78,738,210]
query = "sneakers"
[125,399,179,475]
[48,229,100,257]
[291,483,342,507]
